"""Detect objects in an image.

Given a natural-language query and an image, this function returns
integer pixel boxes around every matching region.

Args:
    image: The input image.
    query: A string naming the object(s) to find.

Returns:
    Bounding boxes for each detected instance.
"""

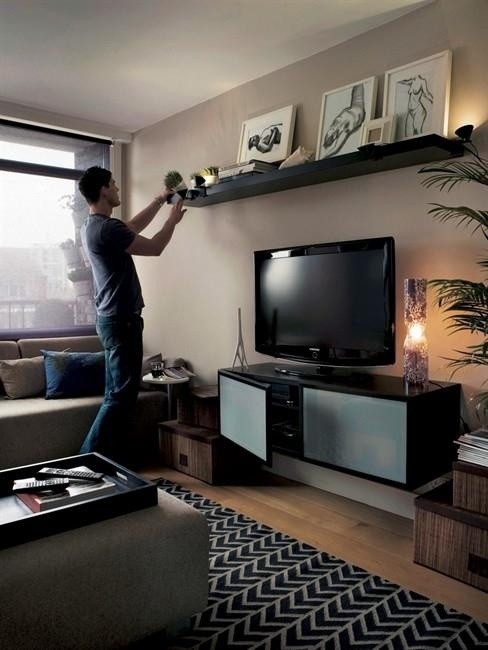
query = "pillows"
[0,347,163,401]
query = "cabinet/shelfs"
[217,361,462,521]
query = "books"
[13,465,117,513]
[215,159,278,184]
[451,428,488,468]
[163,367,198,380]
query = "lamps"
[401,276,429,386]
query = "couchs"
[0,325,166,471]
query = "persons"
[318,83,366,159]
[78,165,191,461]
[398,74,434,137]
[248,122,284,154]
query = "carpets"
[124,475,488,650]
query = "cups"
[149,362,163,378]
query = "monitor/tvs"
[254,236,397,381]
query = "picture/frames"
[236,103,297,166]
[314,49,453,162]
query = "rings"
[174,206,178,209]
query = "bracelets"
[156,197,164,207]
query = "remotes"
[37,466,105,482]
[12,477,71,494]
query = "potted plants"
[163,166,220,193]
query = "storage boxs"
[411,460,488,593]
[156,386,243,486]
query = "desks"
[0,488,212,649]
[141,373,189,420]
